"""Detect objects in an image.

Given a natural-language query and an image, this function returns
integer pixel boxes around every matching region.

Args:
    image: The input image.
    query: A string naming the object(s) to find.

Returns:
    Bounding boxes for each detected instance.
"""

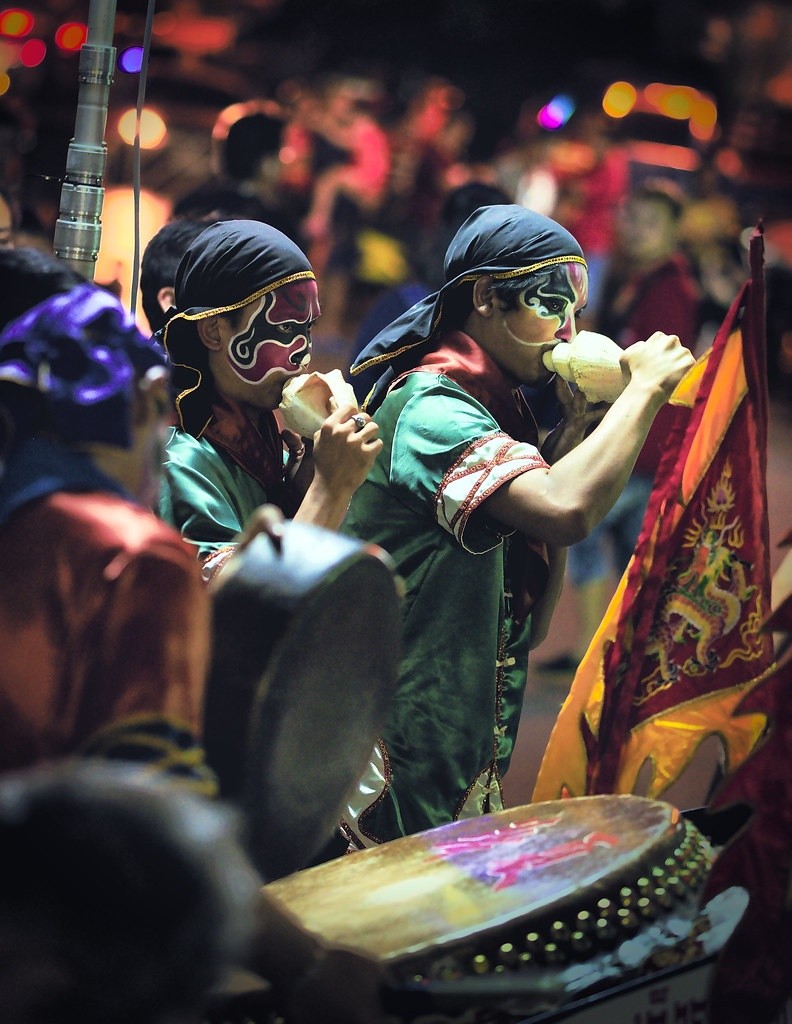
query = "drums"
[206,789,718,1024]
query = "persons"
[149,219,384,584]
[0,244,270,1024]
[339,205,696,855]
[207,103,708,675]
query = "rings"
[350,416,366,428]
